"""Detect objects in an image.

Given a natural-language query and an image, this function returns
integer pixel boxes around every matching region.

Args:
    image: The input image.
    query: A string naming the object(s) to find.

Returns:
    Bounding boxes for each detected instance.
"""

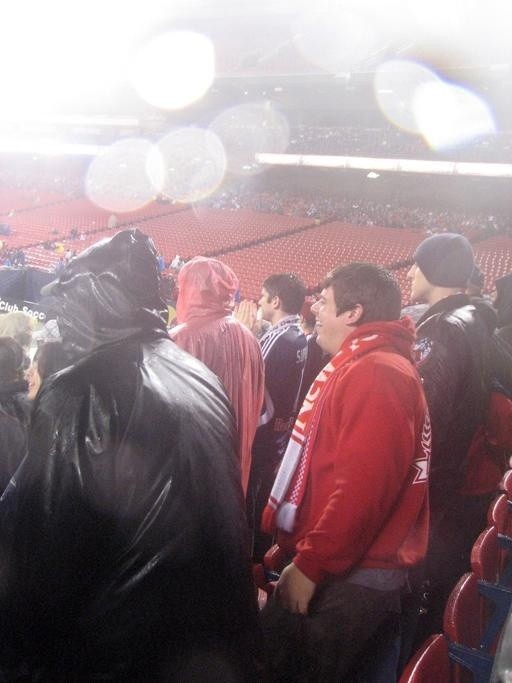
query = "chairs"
[399,456,512,683]
[0,188,512,309]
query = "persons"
[1,229,512,683]
[153,79,511,238]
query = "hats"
[411,232,475,288]
